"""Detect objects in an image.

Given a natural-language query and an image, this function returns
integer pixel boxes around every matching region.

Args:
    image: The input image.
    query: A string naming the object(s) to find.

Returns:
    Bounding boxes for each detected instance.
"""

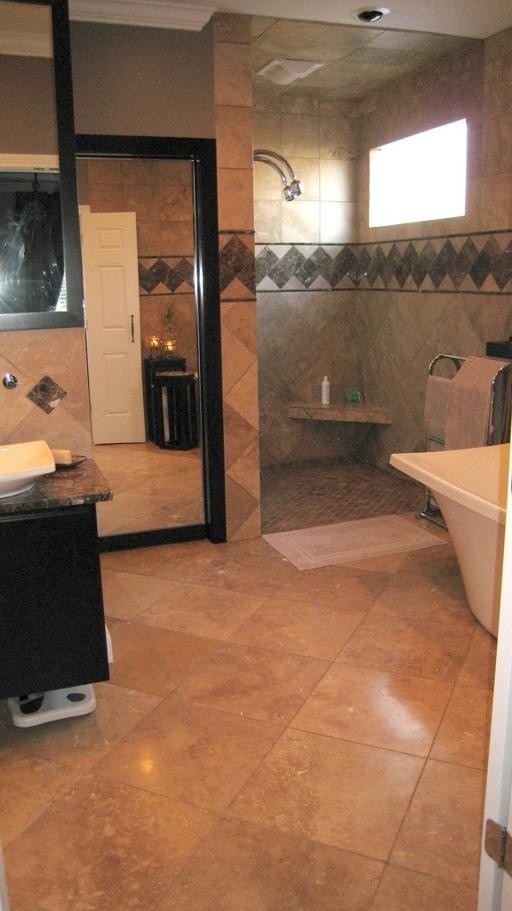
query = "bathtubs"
[389,442,511,641]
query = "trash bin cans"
[153,372,198,451]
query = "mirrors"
[0,0,85,331]
[0,133,226,555]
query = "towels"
[446,355,511,449]
[424,376,450,429]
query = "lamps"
[147,334,177,359]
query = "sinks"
[0,438,55,497]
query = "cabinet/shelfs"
[0,503,113,728]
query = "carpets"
[262,513,448,572]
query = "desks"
[144,356,186,443]
